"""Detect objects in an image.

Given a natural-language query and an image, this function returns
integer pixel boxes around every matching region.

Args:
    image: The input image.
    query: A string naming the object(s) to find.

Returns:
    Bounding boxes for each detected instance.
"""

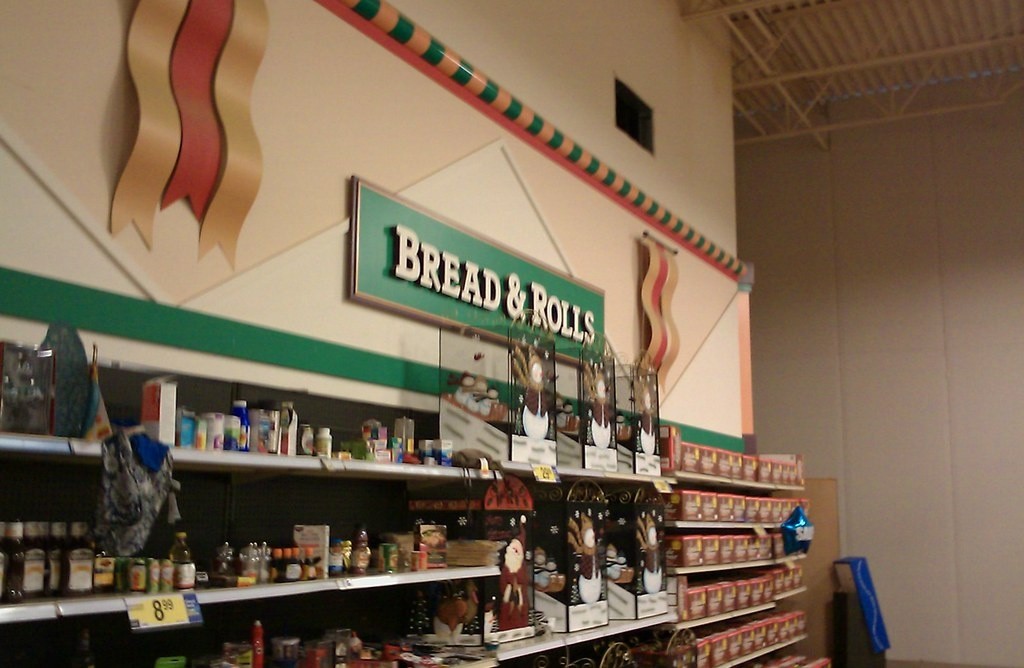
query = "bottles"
[4,338,371,666]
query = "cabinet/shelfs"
[0,429,507,668]
[409,461,677,661]
[650,470,810,668]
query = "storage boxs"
[412,309,668,648]
[657,426,833,668]
